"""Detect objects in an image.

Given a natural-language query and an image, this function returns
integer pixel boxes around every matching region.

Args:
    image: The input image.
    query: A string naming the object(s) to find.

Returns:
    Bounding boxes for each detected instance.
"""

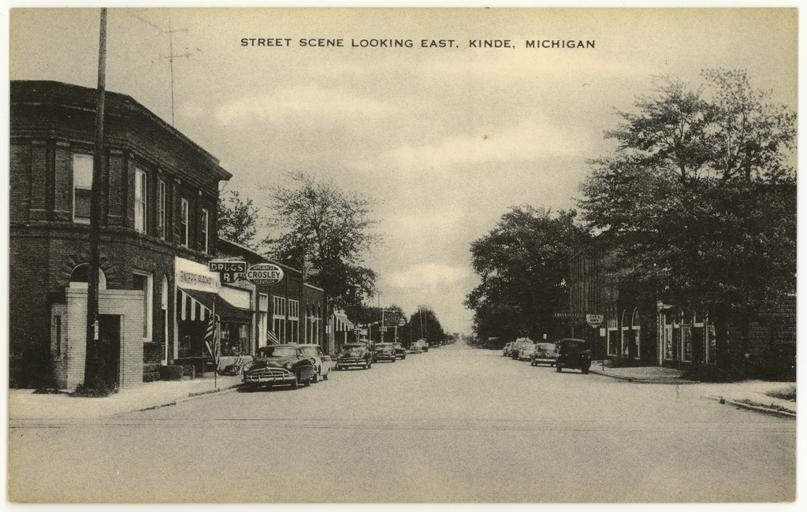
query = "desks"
[216,355,253,376]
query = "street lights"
[380,308,395,342]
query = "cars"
[336,341,372,371]
[392,340,429,360]
[372,343,395,364]
[502,337,534,361]
[241,344,313,390]
[529,341,557,367]
[291,343,332,382]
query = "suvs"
[554,338,590,374]
